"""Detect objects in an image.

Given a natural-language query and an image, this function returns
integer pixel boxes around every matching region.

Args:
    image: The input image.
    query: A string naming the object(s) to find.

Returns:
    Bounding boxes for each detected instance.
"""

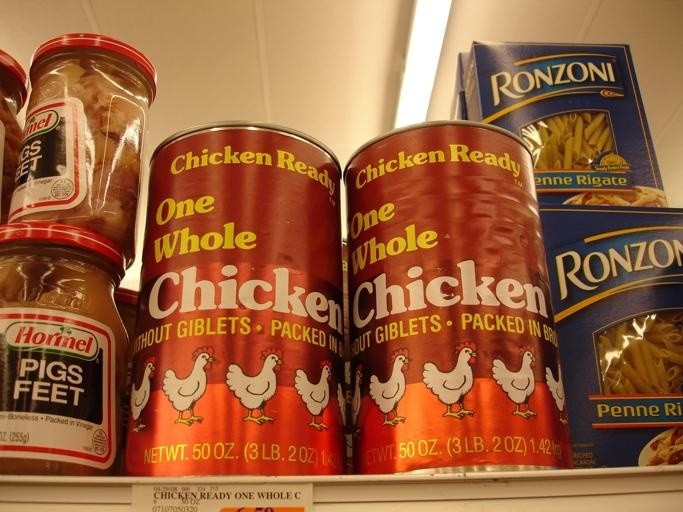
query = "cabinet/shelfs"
[0,468,683,512]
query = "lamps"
[390,1,452,128]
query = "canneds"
[7,33,159,272]
[0,220,131,475]
[0,50,30,224]
[124,120,349,478]
[343,121,574,475]
